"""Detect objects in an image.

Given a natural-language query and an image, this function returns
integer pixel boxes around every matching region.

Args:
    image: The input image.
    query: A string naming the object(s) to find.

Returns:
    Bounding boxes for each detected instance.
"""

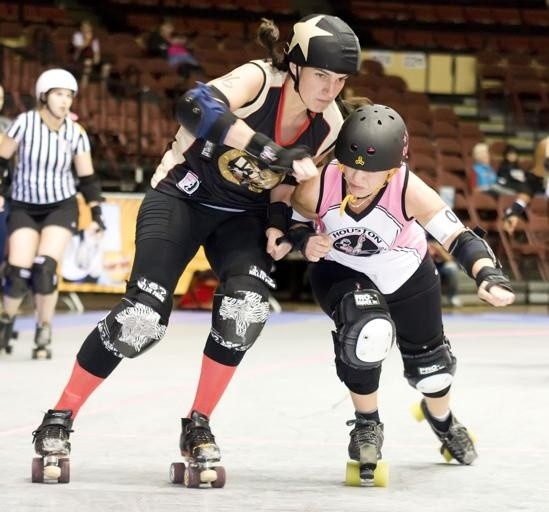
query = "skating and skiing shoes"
[31,408,74,482]
[410,398,479,464]
[345,412,389,487]
[170,411,226,488]
[32,321,52,359]
[0,312,18,353]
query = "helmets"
[283,13,362,74]
[334,104,409,171]
[35,69,78,102]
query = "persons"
[84,110,126,181]
[2,14,202,99]
[1,68,107,358]
[30,12,362,464]
[290,101,516,467]
[471,134,549,235]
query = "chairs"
[1,1,548,284]
